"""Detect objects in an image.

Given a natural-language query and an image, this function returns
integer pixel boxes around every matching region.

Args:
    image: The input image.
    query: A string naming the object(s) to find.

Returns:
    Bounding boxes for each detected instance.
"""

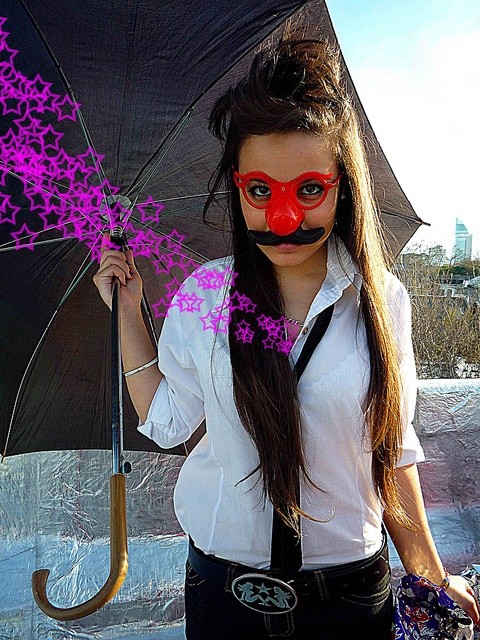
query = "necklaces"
[284,315,305,327]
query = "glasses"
[232,170,343,210]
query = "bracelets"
[122,357,159,377]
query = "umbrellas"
[0,1,431,623]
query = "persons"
[91,21,479,638]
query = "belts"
[187,544,389,616]
